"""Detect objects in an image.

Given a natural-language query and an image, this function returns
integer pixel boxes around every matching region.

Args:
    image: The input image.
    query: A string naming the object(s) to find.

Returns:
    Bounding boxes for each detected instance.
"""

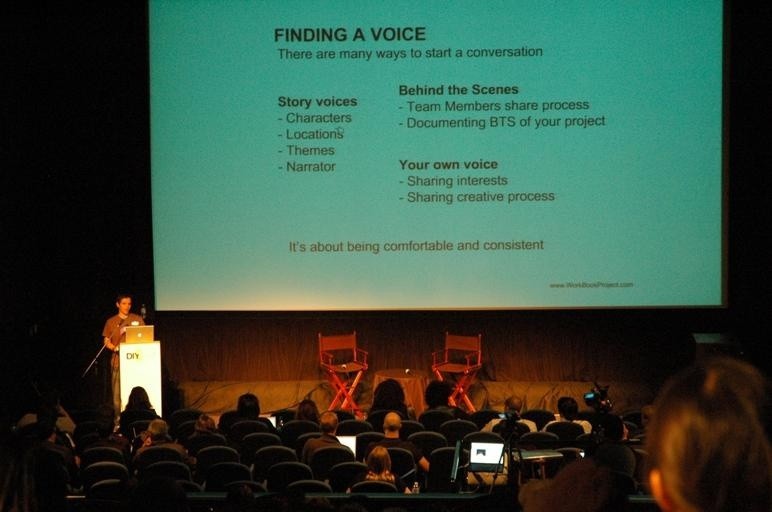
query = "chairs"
[17,405,654,511]
[431,332,484,412]
[318,330,370,415]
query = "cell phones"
[498,414,507,418]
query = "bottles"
[141,304,146,318]
[413,481,419,493]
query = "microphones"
[116,315,127,327]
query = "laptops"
[469,442,504,473]
[267,416,277,429]
[125,325,156,344]
[334,435,357,457]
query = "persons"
[103,294,146,433]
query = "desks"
[372,369,430,419]
